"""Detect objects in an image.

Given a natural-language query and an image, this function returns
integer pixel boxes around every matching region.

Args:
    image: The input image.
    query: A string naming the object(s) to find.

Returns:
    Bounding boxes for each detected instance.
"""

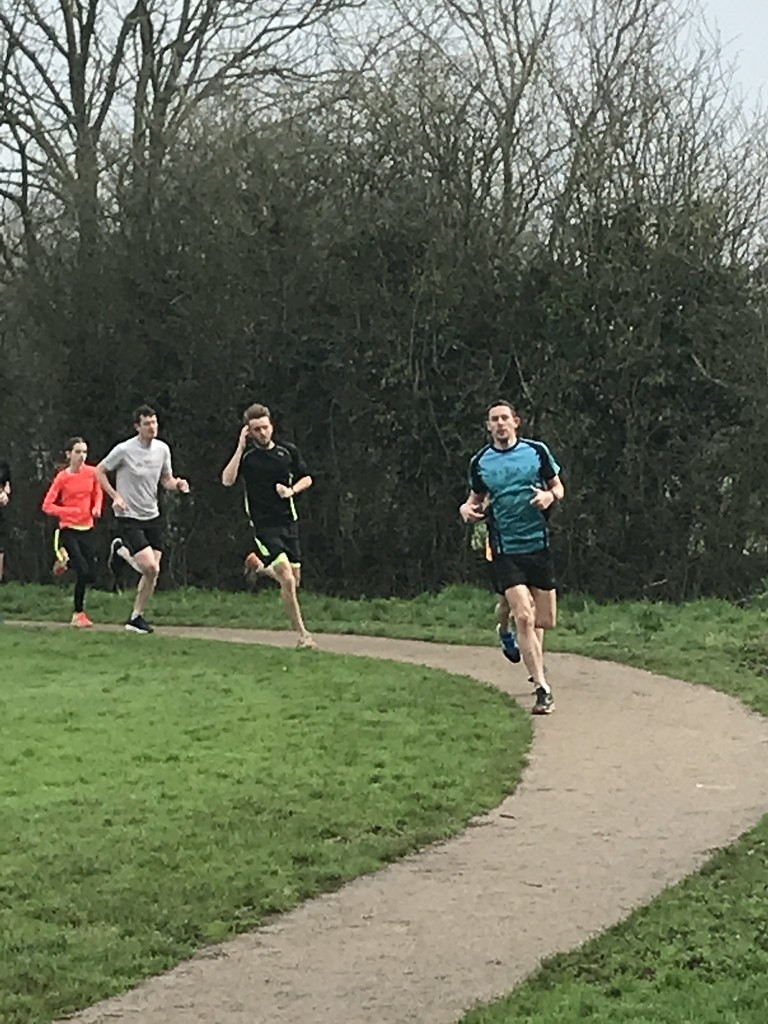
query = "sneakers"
[124,610,154,634]
[106,537,125,576]
[493,623,524,664]
[531,687,556,714]
[52,546,70,577]
[69,611,95,629]
[294,634,330,653]
[242,552,265,594]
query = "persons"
[95,405,190,635]
[42,438,103,627]
[0,458,11,585]
[221,404,319,650]
[460,400,564,714]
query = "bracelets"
[550,490,558,504]
[290,486,296,494]
[2,490,9,497]
